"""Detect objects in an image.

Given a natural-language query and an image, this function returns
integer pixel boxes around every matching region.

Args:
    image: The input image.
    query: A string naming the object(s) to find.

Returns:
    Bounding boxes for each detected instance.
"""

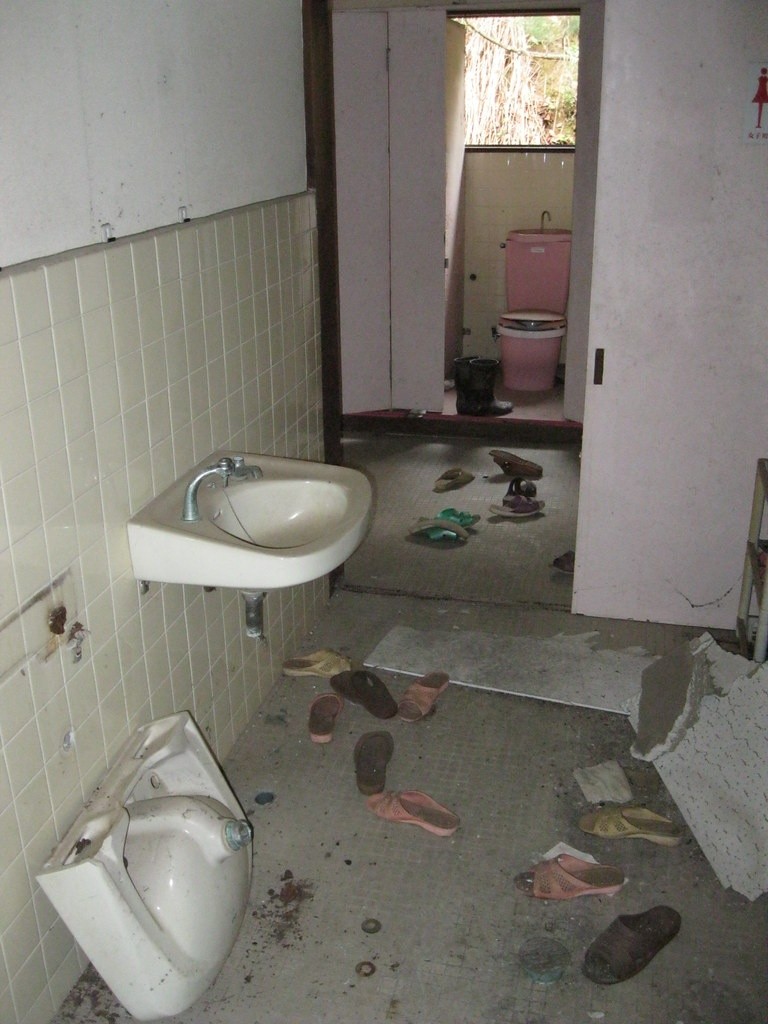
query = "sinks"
[126,449,374,591]
[33,709,255,1022]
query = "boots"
[454,356,513,416]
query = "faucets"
[231,456,264,481]
[180,457,236,522]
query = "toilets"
[496,228,573,392]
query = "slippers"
[583,905,681,985]
[397,670,449,721]
[503,477,536,506]
[409,507,481,545]
[549,551,574,575]
[434,468,474,492]
[309,692,342,743]
[367,789,460,836]
[579,805,683,847]
[330,669,398,720]
[515,853,624,899]
[282,648,361,677]
[490,496,545,517]
[355,730,394,794]
[489,450,542,479]
